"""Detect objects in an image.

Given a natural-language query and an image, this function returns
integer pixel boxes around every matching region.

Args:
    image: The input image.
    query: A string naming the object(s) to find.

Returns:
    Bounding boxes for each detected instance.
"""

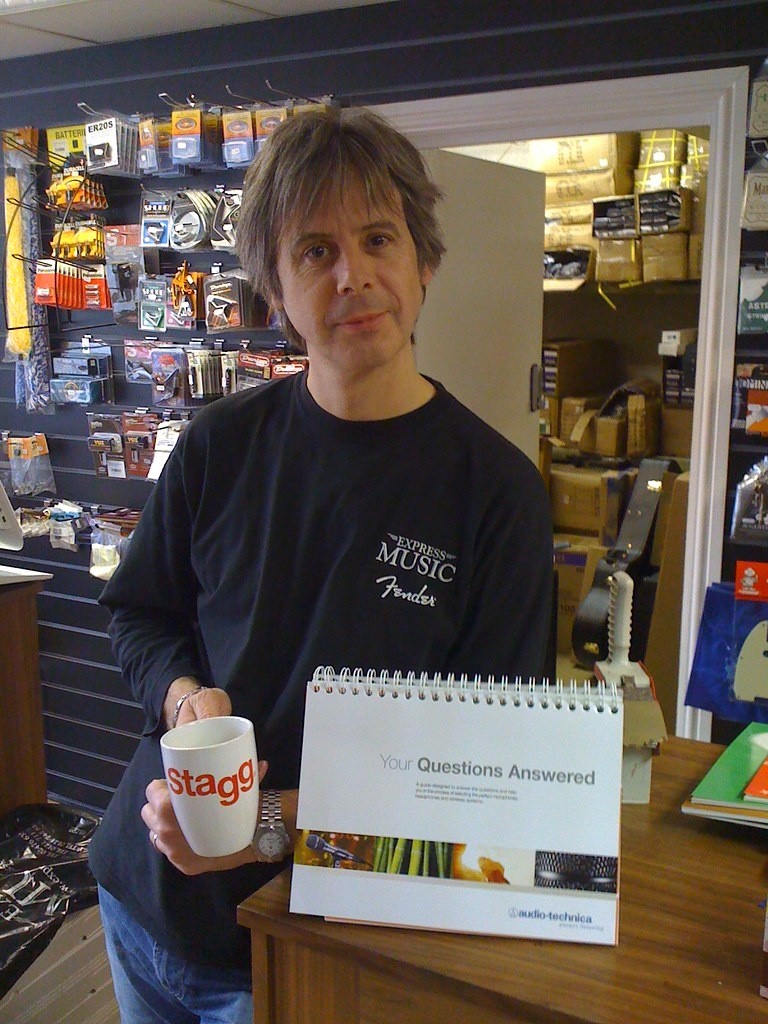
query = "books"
[680,721,768,829]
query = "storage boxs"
[504,129,768,663]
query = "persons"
[86,105,554,1024]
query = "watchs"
[252,789,292,863]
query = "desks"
[236,737,768,1024]
[0,565,53,816]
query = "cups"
[158,715,260,858]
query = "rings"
[153,834,159,850]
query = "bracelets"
[173,685,208,728]
